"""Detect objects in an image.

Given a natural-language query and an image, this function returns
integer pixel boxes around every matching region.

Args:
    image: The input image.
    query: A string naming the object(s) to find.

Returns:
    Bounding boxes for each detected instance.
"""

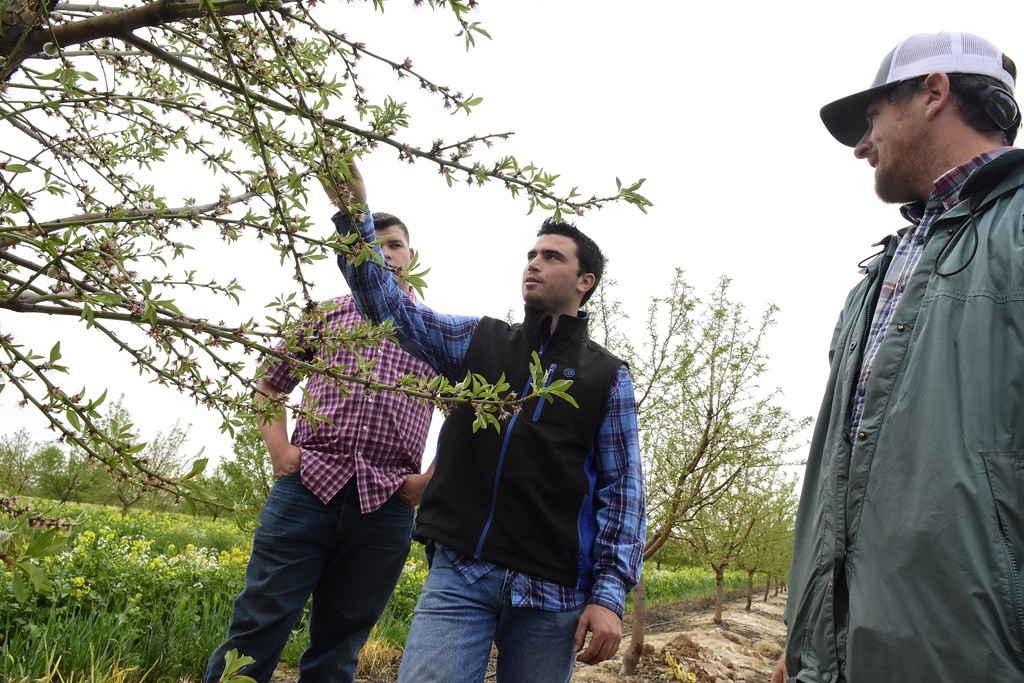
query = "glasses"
[948,75,1022,132]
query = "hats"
[820,31,1016,147]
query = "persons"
[771,31,1024,683]
[317,136,648,683]
[205,211,455,683]
